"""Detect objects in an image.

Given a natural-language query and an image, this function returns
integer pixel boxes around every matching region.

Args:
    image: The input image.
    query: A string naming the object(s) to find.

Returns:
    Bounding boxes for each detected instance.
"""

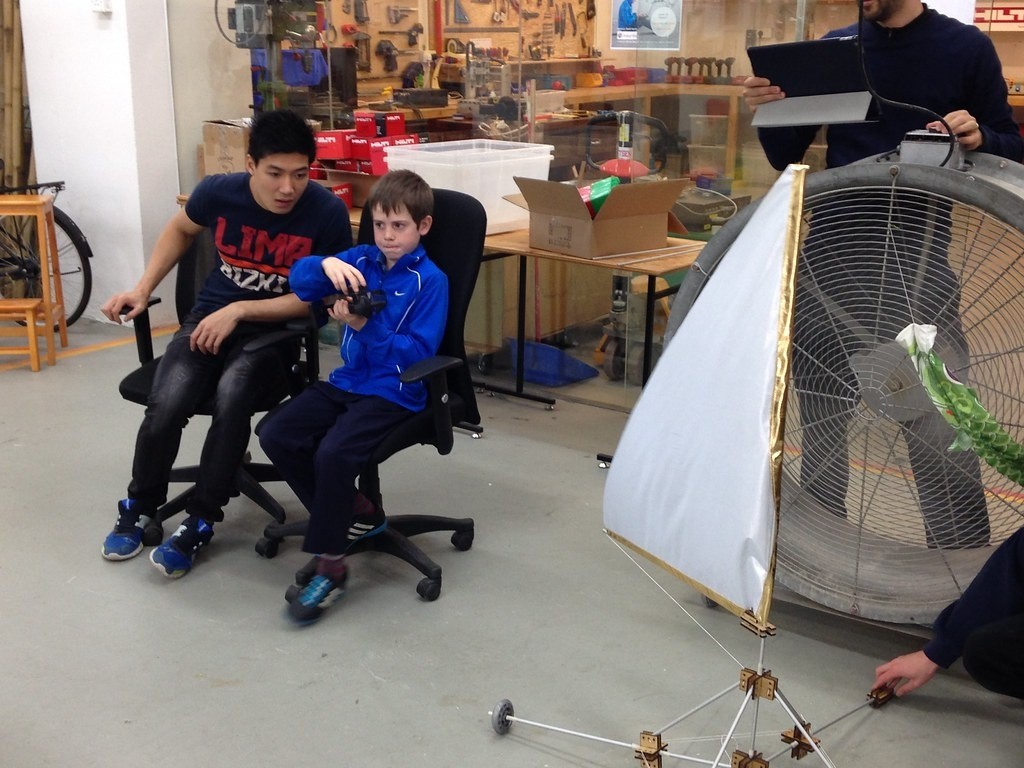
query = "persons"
[619,0,644,29]
[871,522,1024,699]
[101,110,357,582]
[745,0,1012,552]
[258,171,449,621]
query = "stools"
[0,195,68,371]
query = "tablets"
[748,35,883,121]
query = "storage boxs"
[308,163,329,179]
[354,111,406,137]
[351,133,430,160]
[310,178,352,211]
[310,169,387,207]
[203,118,322,175]
[197,144,206,182]
[360,158,389,176]
[334,159,368,173]
[384,139,555,235]
[313,128,355,159]
[501,175,691,259]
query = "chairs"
[119,238,326,544]
[253,190,486,617]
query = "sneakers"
[342,500,388,551]
[150,514,214,579]
[289,564,348,626]
[101,499,157,560]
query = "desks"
[354,83,748,180]
[177,194,707,468]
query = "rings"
[976,123,979,128]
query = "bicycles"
[0,157,95,333]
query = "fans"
[661,148,1024,625]
